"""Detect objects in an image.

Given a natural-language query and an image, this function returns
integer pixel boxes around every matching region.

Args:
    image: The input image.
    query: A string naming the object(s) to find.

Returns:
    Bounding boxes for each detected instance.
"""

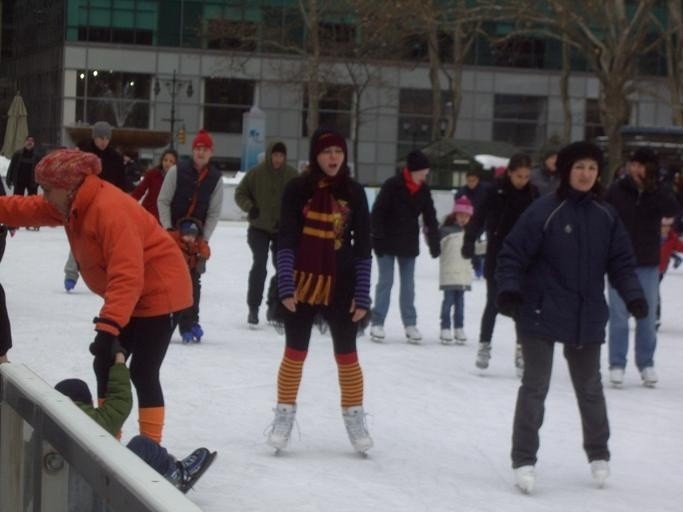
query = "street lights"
[152,68,193,154]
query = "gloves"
[89,331,128,361]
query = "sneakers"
[63,277,77,289]
[339,405,374,452]
[640,365,659,383]
[181,324,203,341]
[514,465,537,490]
[266,402,296,449]
[165,448,209,491]
[590,459,614,483]
[245,304,525,369]
[609,366,625,384]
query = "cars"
[592,124,683,176]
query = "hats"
[467,163,482,178]
[453,195,474,216]
[407,149,431,171]
[92,120,112,139]
[191,127,214,149]
[33,147,103,190]
[314,132,347,155]
[54,379,93,405]
[554,139,606,170]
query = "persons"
[235,125,683,494]
[1,122,223,493]
